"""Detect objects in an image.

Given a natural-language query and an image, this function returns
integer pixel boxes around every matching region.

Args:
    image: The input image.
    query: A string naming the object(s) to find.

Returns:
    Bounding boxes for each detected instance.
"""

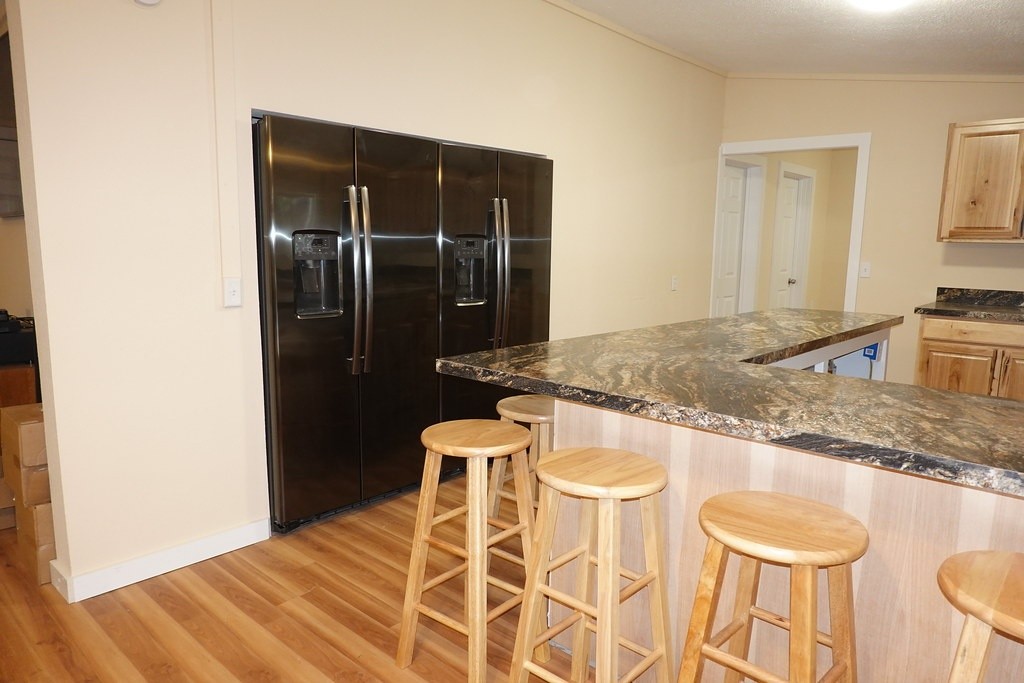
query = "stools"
[936,549,1024,683]
[508,447,674,683]
[487,394,554,574]
[397,419,550,683]
[673,491,869,683]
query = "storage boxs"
[0,402,58,585]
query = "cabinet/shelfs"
[913,314,1024,403]
[936,117,1024,244]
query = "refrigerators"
[256,115,439,535]
[438,140,552,479]
